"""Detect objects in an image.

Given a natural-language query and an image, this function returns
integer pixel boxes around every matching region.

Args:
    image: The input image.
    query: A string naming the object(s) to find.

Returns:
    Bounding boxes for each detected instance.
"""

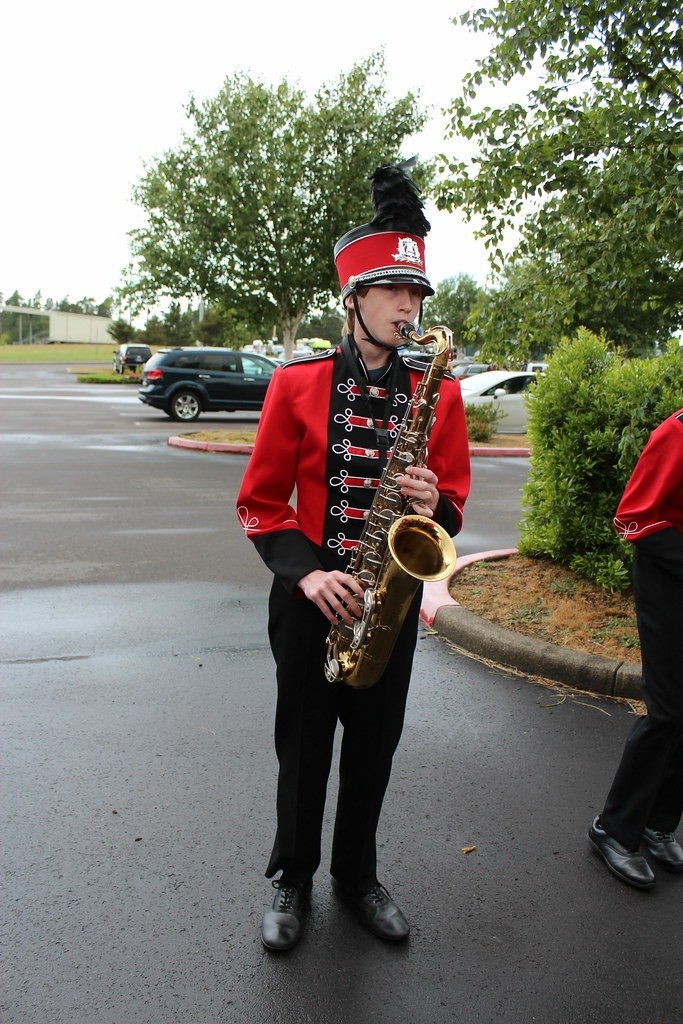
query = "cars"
[264,345,315,364]
[450,356,489,380]
[459,371,546,434]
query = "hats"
[332,163,437,305]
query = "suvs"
[112,344,152,374]
[137,347,280,423]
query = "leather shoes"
[642,826,682,868]
[585,814,656,891]
[330,875,412,946]
[259,865,313,954]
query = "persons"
[236,161,473,955]
[588,406,683,886]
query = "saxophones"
[324,321,457,690]
